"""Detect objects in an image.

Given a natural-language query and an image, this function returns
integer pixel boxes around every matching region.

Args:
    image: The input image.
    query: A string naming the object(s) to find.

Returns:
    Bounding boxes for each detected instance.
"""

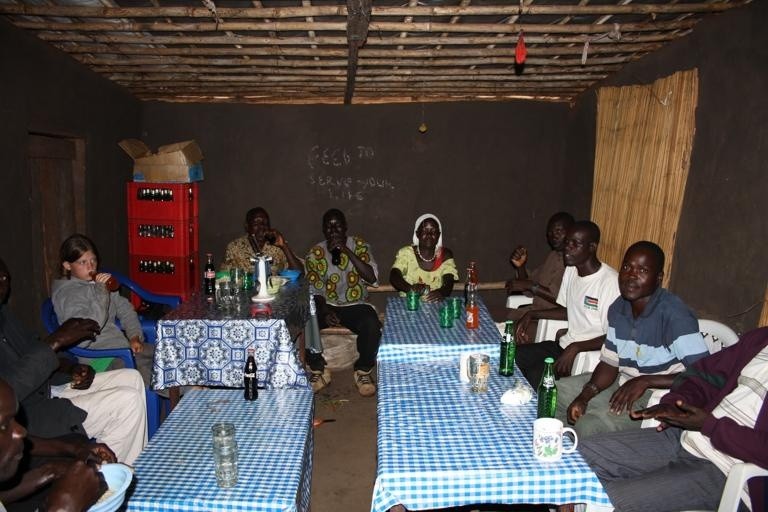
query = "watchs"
[534,281,541,293]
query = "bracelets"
[582,381,601,396]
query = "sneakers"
[354,368,376,396]
[309,367,332,392]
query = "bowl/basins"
[85,463,133,512]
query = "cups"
[213,439,239,487]
[439,303,455,328]
[449,299,462,319]
[532,417,580,463]
[467,353,490,397]
[212,423,235,444]
[217,281,233,312]
[230,266,247,297]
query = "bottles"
[440,305,454,327]
[89,270,118,290]
[466,283,478,328]
[139,260,175,275]
[244,349,258,401]
[244,272,252,288]
[537,358,556,417]
[204,253,216,297]
[499,321,515,376]
[466,260,478,287]
[465,269,475,305]
[451,299,461,318]
[138,188,173,202]
[189,189,193,200]
[333,247,341,264]
[139,224,174,239]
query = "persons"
[508,220,620,389]
[221,205,307,283]
[574,327,768,511]
[503,212,575,322]
[1,380,109,512]
[51,233,209,397]
[301,207,383,396]
[389,213,459,304]
[549,240,709,437]
[0,263,103,443]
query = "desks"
[127,389,313,512]
[370,361,615,512]
[150,283,312,411]
[376,293,503,362]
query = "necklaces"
[416,246,437,262]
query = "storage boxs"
[129,257,201,293]
[128,182,198,220]
[128,221,197,257]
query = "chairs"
[43,270,182,441]
[640,398,768,511]
[545,320,586,375]
[581,319,740,397]
[495,295,546,343]
